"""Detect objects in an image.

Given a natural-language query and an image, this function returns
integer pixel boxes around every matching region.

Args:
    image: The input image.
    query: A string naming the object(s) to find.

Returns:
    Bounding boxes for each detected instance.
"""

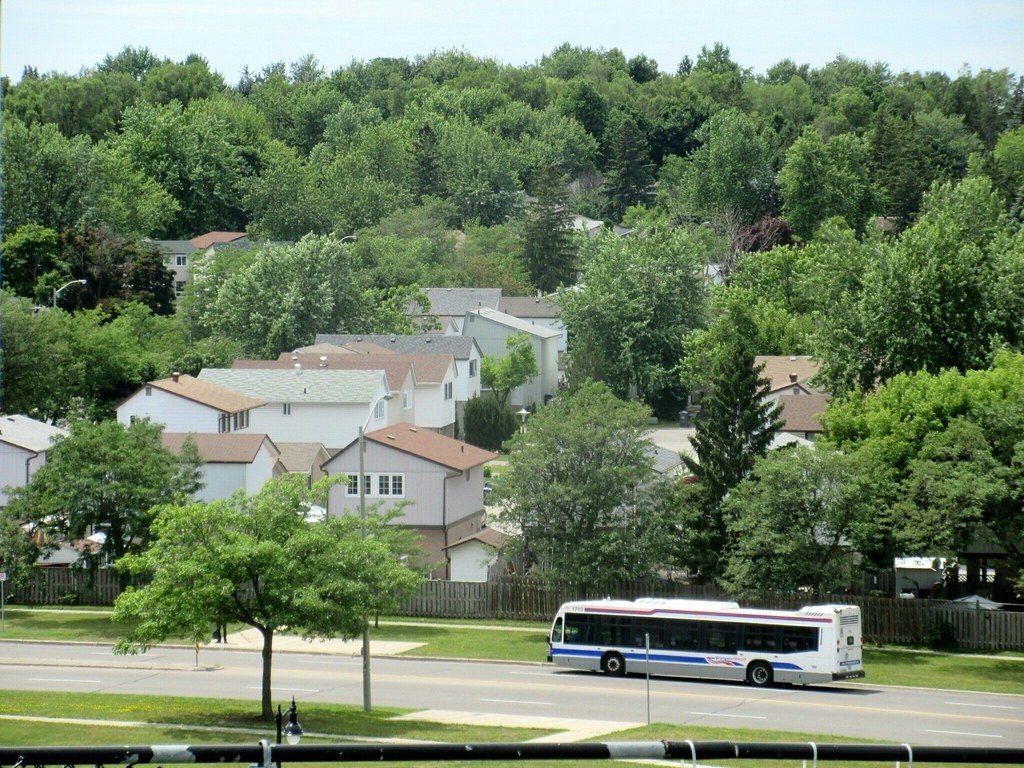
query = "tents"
[947,594,1005,610]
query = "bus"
[546,596,865,690]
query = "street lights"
[52,279,88,306]
